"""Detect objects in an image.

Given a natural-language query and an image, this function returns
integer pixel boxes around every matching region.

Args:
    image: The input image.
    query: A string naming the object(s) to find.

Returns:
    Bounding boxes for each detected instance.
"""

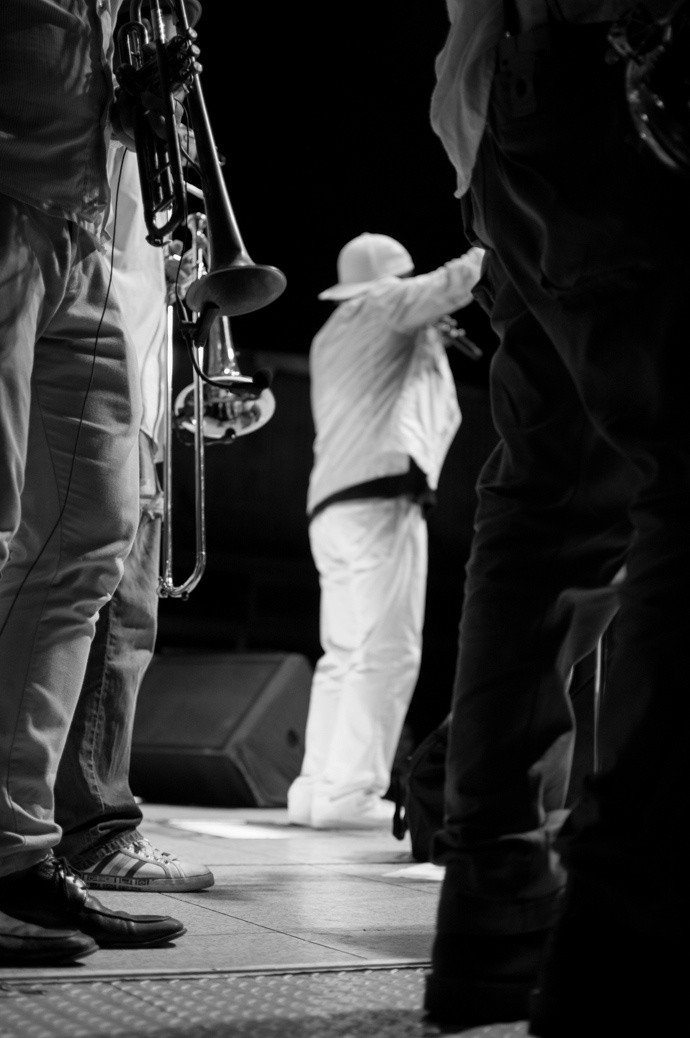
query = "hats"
[318,232,414,300]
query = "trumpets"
[115,0,288,319]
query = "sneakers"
[71,836,214,891]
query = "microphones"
[184,332,263,401]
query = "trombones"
[154,212,271,602]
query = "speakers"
[128,650,317,809]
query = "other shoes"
[288,790,314,825]
[423,914,560,1035]
[531,922,690,1038]
[310,798,406,829]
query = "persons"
[0,0,214,966]
[385,0,690,1038]
[287,232,487,830]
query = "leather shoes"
[0,855,187,949]
[0,910,100,968]
